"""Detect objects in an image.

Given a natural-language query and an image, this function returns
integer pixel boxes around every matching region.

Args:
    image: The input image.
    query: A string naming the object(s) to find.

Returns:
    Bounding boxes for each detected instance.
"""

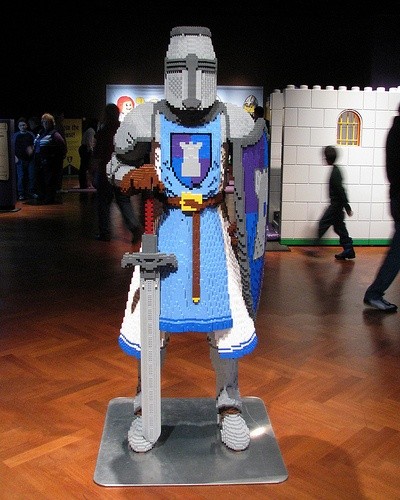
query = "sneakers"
[306,248,320,256]
[363,293,398,312]
[335,249,357,261]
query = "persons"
[79,118,99,190]
[89,104,146,243]
[33,113,68,204]
[105,26,272,453]
[362,103,400,313]
[252,105,271,133]
[305,147,357,260]
[11,118,40,200]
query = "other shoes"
[19,194,25,200]
